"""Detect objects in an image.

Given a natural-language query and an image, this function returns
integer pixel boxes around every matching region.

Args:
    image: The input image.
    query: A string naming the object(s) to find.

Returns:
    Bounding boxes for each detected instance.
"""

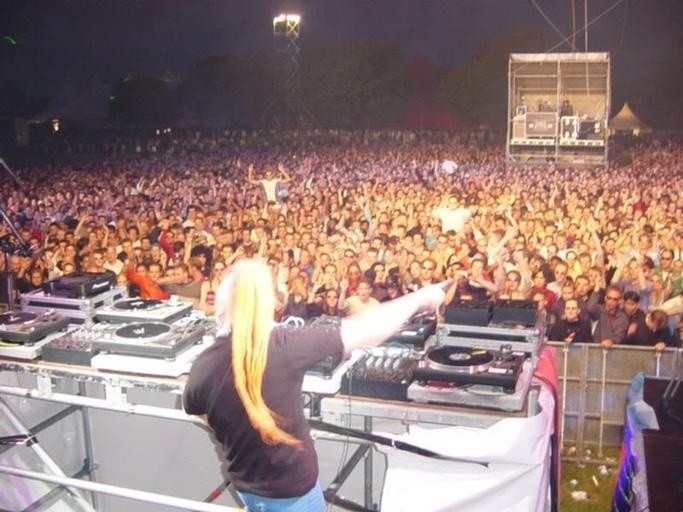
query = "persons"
[0,101,683,351]
[183,260,452,512]
